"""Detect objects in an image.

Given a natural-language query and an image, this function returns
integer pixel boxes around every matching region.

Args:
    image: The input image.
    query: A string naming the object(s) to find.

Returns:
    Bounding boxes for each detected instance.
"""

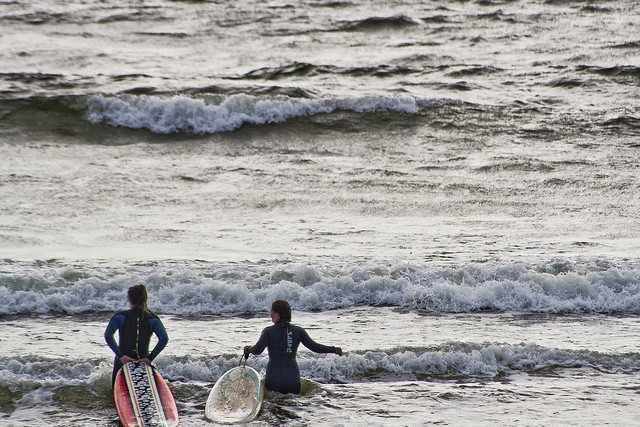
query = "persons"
[244,300,342,394]
[104,284,168,390]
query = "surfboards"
[114,360,179,427]
[205,364,264,424]
